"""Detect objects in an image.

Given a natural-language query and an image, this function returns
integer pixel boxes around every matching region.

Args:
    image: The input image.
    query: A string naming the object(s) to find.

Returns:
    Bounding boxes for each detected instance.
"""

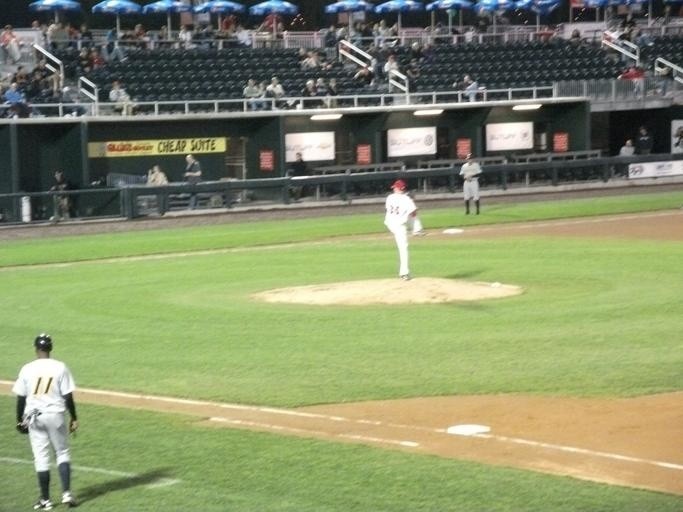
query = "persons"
[146,164,169,215]
[635,125,654,154]
[382,179,421,281]
[0,21,681,119]
[613,139,635,178]
[47,169,74,221]
[286,153,307,203]
[182,154,202,209]
[459,152,483,216]
[11,331,78,511]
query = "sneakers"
[400,274,410,281]
[59,492,75,505]
[32,498,51,511]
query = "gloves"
[413,218,423,235]
[68,420,78,432]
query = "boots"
[474,200,481,215]
[463,199,471,216]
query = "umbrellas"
[24,0,677,34]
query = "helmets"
[33,334,53,352]
[390,179,408,191]
[465,153,475,160]
